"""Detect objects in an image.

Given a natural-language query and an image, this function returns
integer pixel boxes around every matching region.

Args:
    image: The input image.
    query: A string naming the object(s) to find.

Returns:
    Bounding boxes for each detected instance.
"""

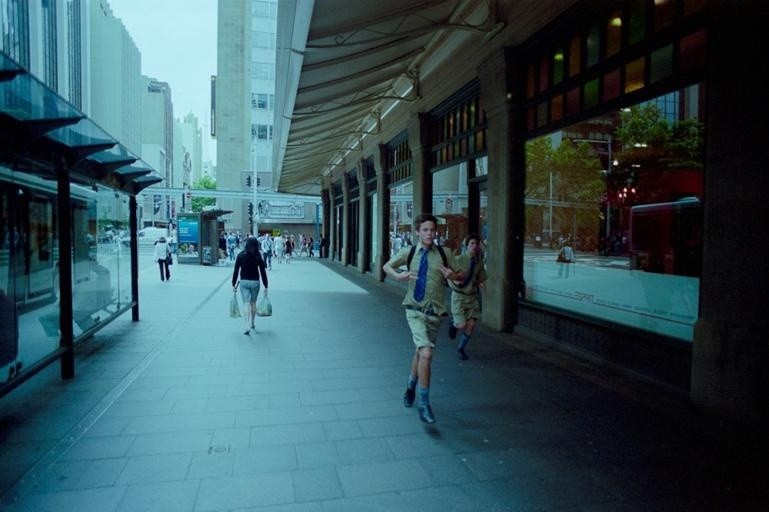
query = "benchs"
[40,297,119,334]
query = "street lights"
[573,136,615,257]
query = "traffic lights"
[248,218,252,224]
[257,176,261,187]
[245,175,251,188]
[248,202,253,216]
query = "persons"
[6,225,20,251]
[386,225,446,257]
[40,227,101,354]
[86,231,93,248]
[534,233,625,280]
[189,242,194,255]
[217,230,326,270]
[153,237,174,281]
[382,212,465,425]
[170,224,177,255]
[232,236,269,334]
[448,232,488,359]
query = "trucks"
[139,226,168,242]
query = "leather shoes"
[448,320,457,340]
[403,376,417,408]
[417,403,435,423]
[456,346,471,361]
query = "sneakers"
[244,326,255,335]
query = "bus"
[627,197,700,279]
[0,164,98,315]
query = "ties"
[414,248,429,302]
[460,257,475,288]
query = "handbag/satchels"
[258,295,271,316]
[167,256,172,265]
